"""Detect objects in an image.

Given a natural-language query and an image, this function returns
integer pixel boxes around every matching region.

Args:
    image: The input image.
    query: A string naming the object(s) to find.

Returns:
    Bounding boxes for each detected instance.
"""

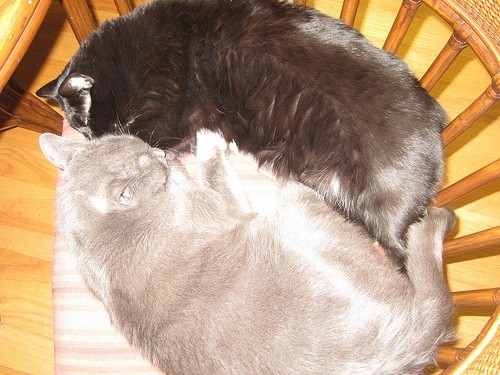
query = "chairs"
[56,1,500,375]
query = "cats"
[36,0,447,273]
[38,126,458,375]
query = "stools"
[0,0,64,136]
